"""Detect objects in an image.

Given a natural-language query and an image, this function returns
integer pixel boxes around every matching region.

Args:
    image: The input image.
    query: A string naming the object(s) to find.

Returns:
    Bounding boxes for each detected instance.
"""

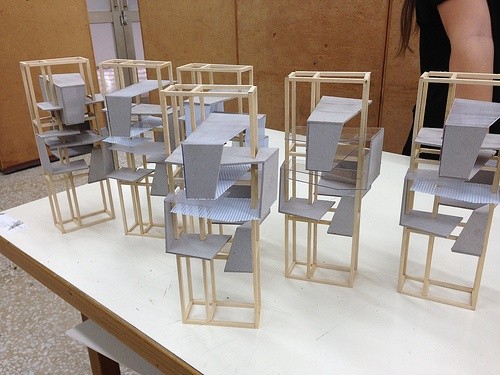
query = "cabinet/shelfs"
[22,54,500,332]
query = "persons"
[392,0,499,163]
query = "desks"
[1,129,500,374]
